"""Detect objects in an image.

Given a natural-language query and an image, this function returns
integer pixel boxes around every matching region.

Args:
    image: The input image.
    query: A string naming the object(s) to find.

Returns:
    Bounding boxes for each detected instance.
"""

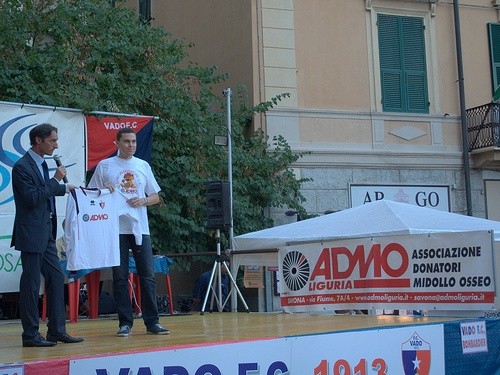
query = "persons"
[10,124,84,347]
[88,128,171,335]
[193,255,230,311]
[55,219,66,261]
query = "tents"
[232,199,500,318]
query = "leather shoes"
[47,333,81,343]
[23,334,56,347]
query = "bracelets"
[144,198,148,204]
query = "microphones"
[53,155,68,183]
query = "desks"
[41,253,173,324]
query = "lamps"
[214,134,228,146]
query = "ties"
[41,160,54,215]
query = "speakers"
[205,180,232,229]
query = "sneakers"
[147,324,170,335]
[117,325,132,336]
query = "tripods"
[200,229,251,315]
